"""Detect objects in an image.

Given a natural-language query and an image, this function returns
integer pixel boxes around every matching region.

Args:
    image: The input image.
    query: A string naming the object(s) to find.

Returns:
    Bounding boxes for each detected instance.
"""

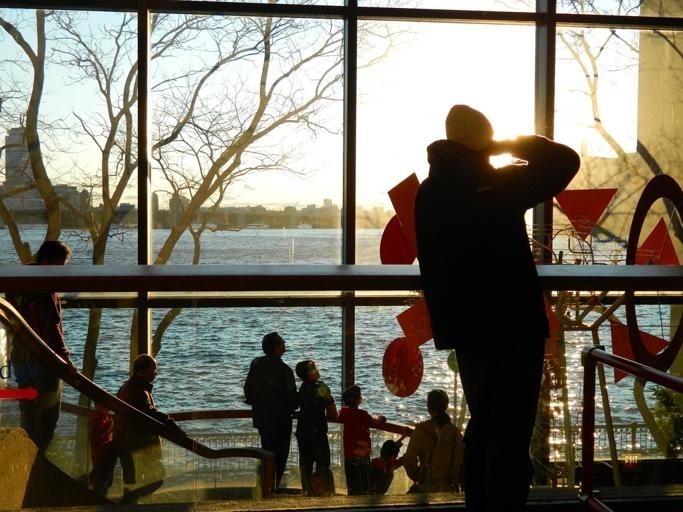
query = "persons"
[111,354,187,505]
[79,401,118,499]
[414,105,580,512]
[11,240,77,456]
[244,332,464,500]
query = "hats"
[443,104,494,152]
